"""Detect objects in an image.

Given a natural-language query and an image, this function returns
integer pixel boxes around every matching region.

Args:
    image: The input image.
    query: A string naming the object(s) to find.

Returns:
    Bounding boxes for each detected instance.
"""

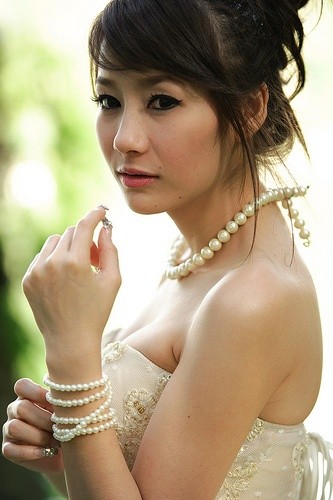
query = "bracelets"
[43,377,117,442]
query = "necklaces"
[161,183,310,282]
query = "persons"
[0,1,328,500]
